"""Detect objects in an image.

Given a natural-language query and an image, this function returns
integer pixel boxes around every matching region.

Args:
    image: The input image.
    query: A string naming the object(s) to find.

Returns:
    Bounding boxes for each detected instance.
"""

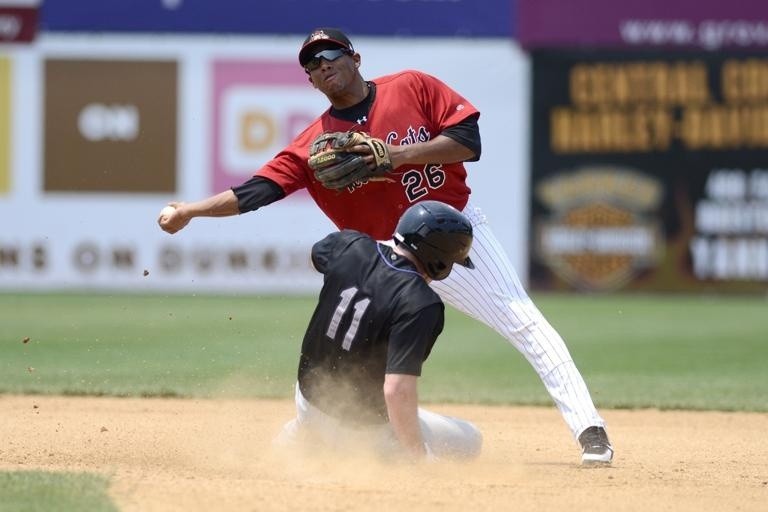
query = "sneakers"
[580,425,613,466]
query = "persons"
[156,26,617,466]
[274,199,484,464]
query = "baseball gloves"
[308,130,392,190]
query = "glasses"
[303,48,350,72]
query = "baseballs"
[160,205,176,220]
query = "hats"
[299,28,354,68]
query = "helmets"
[394,200,474,280]
